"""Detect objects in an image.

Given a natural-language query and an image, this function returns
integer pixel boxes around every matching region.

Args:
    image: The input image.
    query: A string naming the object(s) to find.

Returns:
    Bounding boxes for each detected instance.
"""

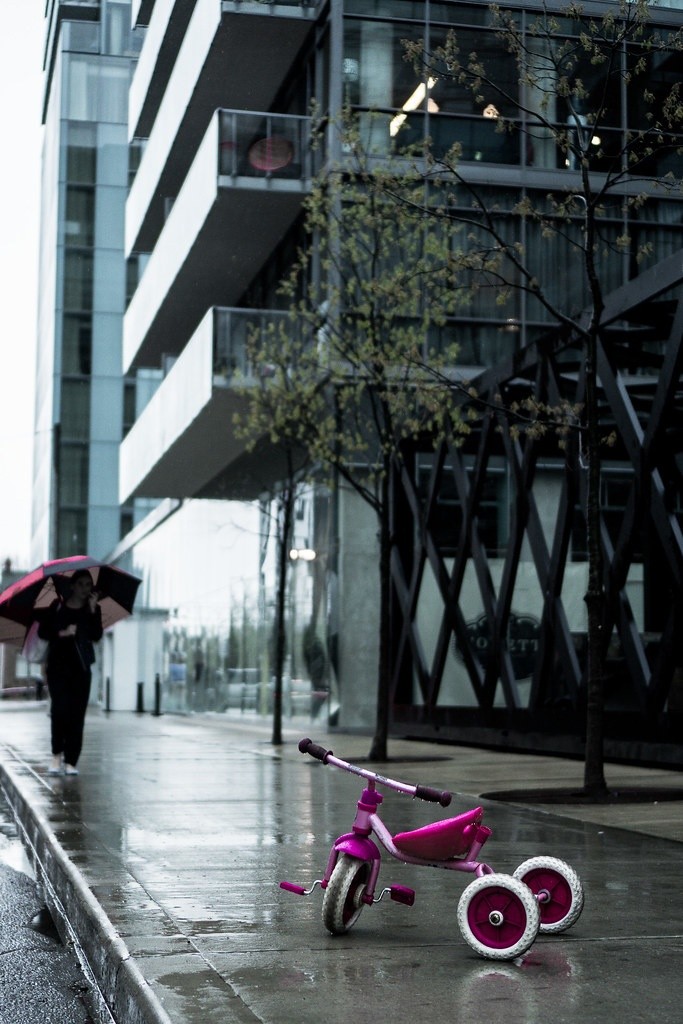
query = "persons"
[37,569,103,775]
[36,680,43,700]
[168,652,186,713]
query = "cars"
[208,667,275,713]
[292,679,314,711]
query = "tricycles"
[278,738,585,962]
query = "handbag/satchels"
[21,621,48,664]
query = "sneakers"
[48,755,62,772]
[64,765,78,774]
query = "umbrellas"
[0,555,141,642]
[31,673,44,681]
